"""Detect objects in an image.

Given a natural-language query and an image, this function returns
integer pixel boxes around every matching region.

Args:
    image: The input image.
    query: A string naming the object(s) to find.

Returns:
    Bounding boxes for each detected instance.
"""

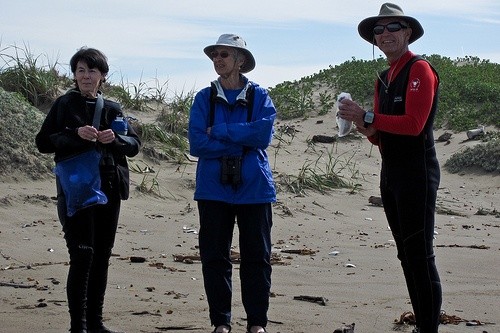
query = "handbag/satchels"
[54,150,108,217]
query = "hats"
[203,34,256,73]
[357,2,424,47]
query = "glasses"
[373,22,408,35]
[210,50,239,58]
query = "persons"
[35,48,142,333]
[338,2,443,333]
[188,33,277,333]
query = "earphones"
[407,31,411,36]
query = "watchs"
[363,111,376,128]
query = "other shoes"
[212,326,232,333]
[247,324,268,333]
[86,325,116,333]
